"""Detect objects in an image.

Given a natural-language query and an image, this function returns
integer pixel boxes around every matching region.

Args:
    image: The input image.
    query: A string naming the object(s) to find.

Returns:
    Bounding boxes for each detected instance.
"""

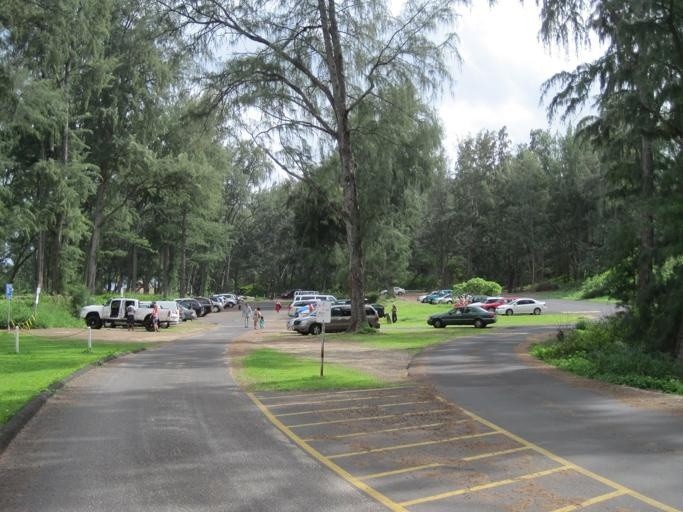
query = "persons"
[252,307,261,330]
[275,299,280,312]
[126,302,135,331]
[259,316,264,329]
[149,303,159,332]
[391,303,397,323]
[240,301,252,328]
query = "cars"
[417,289,547,328]
[280,289,384,335]
[79,293,238,332]
[380,287,406,295]
